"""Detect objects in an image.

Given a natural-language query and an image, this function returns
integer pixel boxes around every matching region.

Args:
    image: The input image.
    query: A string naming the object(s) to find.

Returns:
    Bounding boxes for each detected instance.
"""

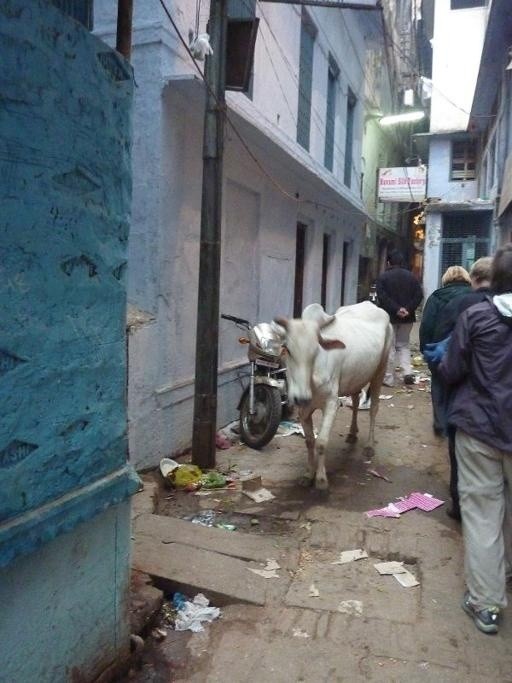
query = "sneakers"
[462,590,499,634]
[404,375,414,385]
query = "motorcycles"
[221,314,298,450]
[368,281,378,302]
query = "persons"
[376,251,423,387]
[418,264,471,434]
[438,243,510,634]
[425,255,495,522]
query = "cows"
[271,298,396,493]
